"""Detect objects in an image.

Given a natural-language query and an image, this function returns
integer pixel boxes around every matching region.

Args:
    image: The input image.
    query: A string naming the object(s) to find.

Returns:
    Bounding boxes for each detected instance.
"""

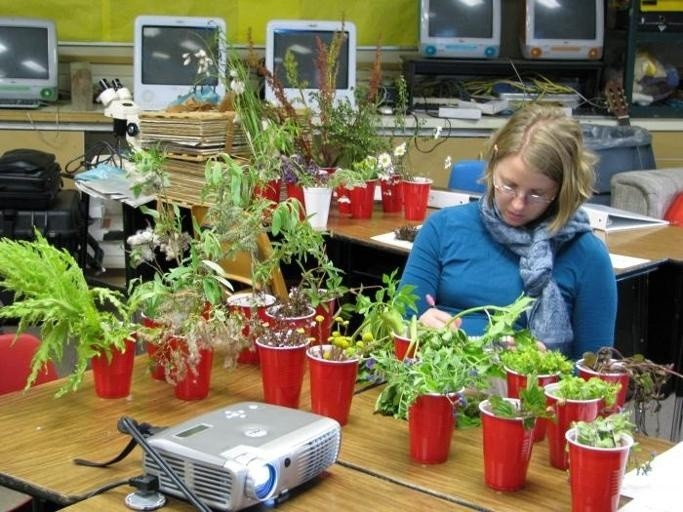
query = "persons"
[396,104,619,377]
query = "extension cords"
[438,105,482,120]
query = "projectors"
[118,395,342,512]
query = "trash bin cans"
[573,123,656,208]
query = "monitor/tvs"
[518,0,606,60]
[0,17,59,103]
[133,14,227,110]
[419,0,502,58]
[265,20,358,107]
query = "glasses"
[492,166,558,205]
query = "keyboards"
[0,99,42,110]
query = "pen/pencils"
[425,293,438,310]
